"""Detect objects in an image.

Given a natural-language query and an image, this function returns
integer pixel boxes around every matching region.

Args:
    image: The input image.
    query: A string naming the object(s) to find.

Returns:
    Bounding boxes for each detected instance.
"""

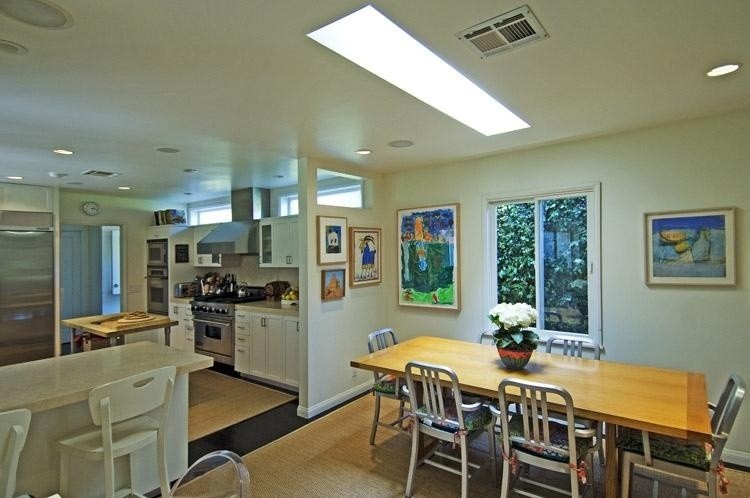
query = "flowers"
[483,302,544,372]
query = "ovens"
[146,267,168,314]
[191,304,233,357]
[147,242,166,266]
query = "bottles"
[224,272,235,294]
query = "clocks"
[80,200,101,216]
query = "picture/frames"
[396,201,462,313]
[348,225,383,290]
[642,206,737,288]
[319,268,346,302]
[315,215,349,266]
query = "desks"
[0,340,215,498]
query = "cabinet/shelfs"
[187,224,236,269]
[234,302,301,394]
[143,221,191,350]
[256,215,302,270]
[168,296,197,352]
[0,179,63,366]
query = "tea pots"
[236,281,251,298]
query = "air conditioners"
[452,3,552,64]
[78,168,122,181]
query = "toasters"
[175,284,191,297]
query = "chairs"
[53,365,178,498]
[477,327,501,349]
[400,360,499,497]
[162,449,255,498]
[0,406,35,498]
[615,373,748,497]
[544,335,605,466]
[364,326,421,446]
[494,379,596,498]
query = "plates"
[117,314,155,322]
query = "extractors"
[194,189,270,255]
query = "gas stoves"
[207,286,267,304]
